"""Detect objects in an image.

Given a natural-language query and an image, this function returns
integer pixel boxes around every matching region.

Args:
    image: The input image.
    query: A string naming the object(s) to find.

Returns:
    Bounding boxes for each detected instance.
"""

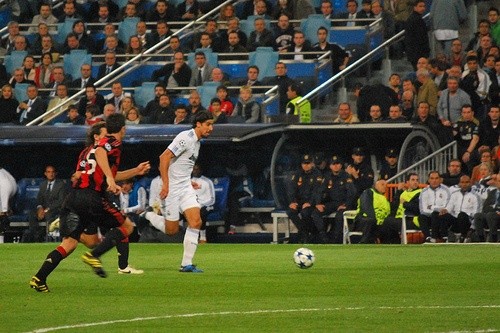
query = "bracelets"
[466,150,472,154]
[341,64,346,66]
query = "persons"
[0,168,17,244]
[462,136,500,200]
[438,175,482,244]
[288,145,375,244]
[97,164,165,245]
[474,173,500,243]
[378,149,399,181]
[330,0,500,164]
[442,159,468,187]
[418,171,451,242]
[0,0,356,127]
[394,173,424,246]
[30,112,151,292]
[137,109,214,274]
[353,178,397,246]
[287,82,311,124]
[27,165,65,243]
[191,164,216,244]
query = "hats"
[387,148,397,159]
[353,147,366,155]
[314,152,324,164]
[329,155,344,164]
[302,154,312,163]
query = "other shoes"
[421,232,472,243]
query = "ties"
[107,67,110,75]
[47,184,51,193]
[83,81,86,85]
[115,98,119,112]
[20,100,31,123]
[199,70,201,86]
[50,84,57,95]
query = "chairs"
[9,174,276,244]
[0,0,382,116]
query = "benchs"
[270,208,408,245]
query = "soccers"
[292,247,315,270]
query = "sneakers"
[92,266,106,279]
[30,276,49,292]
[118,265,143,274]
[81,251,103,268]
[179,264,204,272]
